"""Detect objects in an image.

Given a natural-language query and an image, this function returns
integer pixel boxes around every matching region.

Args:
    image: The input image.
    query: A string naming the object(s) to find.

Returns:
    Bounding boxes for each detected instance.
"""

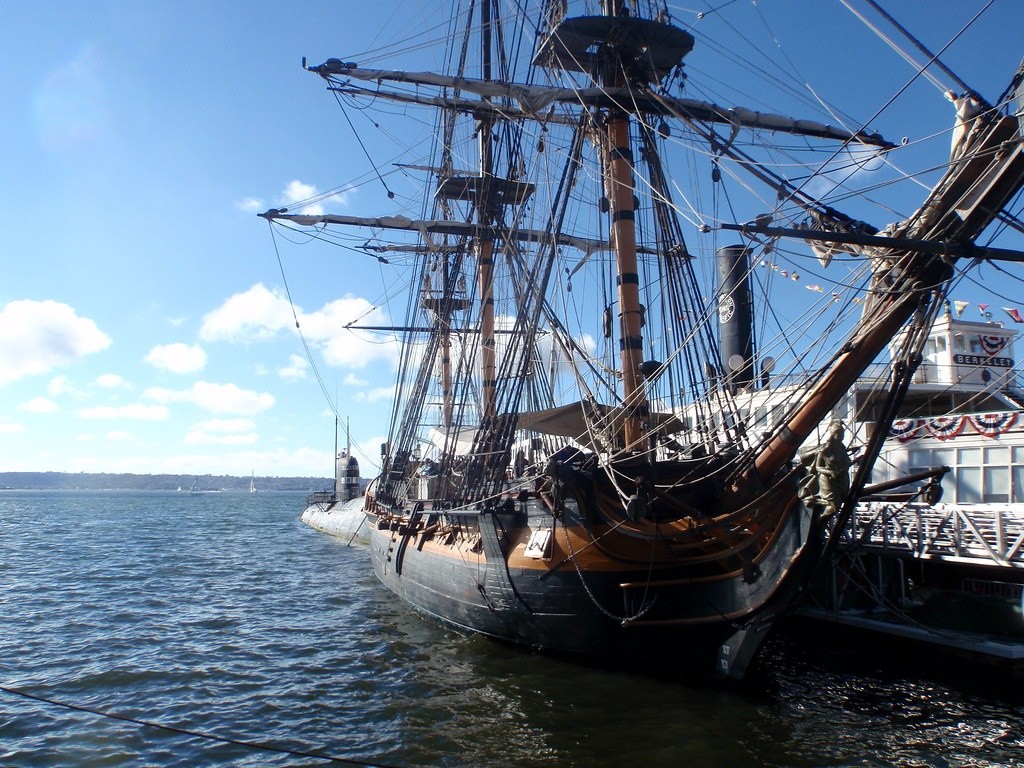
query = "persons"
[814,421,850,524]
[514,448,530,479]
[420,457,438,475]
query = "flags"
[1002,308,1024,323]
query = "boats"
[646,242,1024,661]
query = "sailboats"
[255,0,1023,643]
